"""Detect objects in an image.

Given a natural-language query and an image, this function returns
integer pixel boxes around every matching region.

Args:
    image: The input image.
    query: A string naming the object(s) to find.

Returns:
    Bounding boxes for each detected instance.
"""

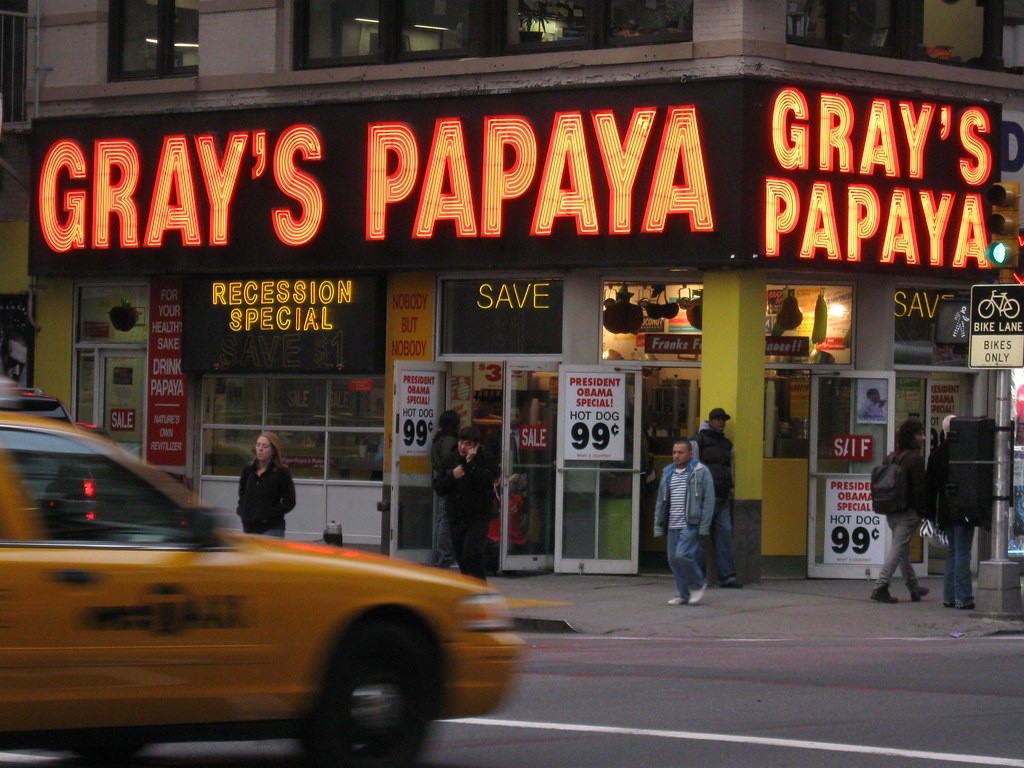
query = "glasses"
[7,354,26,371]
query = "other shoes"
[719,578,743,588]
[957,603,975,610]
[668,597,687,605]
[688,583,707,604]
[943,602,955,607]
[871,588,898,603]
[912,587,929,602]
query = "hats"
[709,409,730,420]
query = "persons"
[858,388,886,420]
[653,440,715,604]
[435,427,494,586]
[689,408,744,589]
[430,410,460,570]
[236,432,296,539]
[924,415,992,609]
[628,424,657,509]
[871,420,929,604]
[486,400,540,474]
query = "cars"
[0,406,524,767]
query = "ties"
[510,432,519,474]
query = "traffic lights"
[982,177,1023,277]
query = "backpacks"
[870,450,913,515]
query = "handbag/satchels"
[919,518,949,549]
[441,458,478,495]
